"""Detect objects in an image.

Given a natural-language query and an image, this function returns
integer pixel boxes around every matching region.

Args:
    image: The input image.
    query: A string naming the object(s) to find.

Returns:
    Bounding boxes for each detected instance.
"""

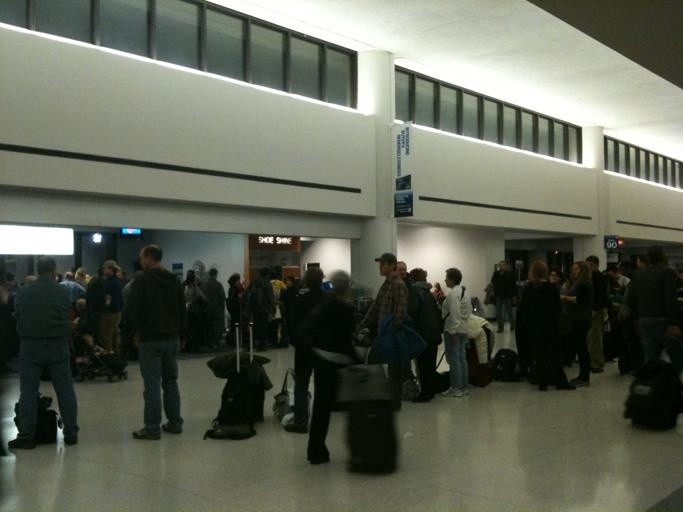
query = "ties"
[119,228,143,237]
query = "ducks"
[335,408,402,477]
[218,363,267,425]
[619,355,680,433]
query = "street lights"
[273,368,313,428]
[331,361,402,406]
[13,392,62,444]
[403,347,517,402]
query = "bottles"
[103,259,123,270]
[373,252,398,264]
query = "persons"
[2,260,227,384]
[602,246,682,367]
[306,269,357,465]
[225,266,305,349]
[516,255,611,391]
[353,252,408,412]
[127,246,188,440]
[8,256,80,450]
[396,261,495,403]
[284,266,329,433]
[491,259,519,334]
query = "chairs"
[441,386,464,398]
[132,427,161,440]
[64,436,78,445]
[161,422,184,434]
[568,376,592,387]
[285,425,330,466]
[7,438,36,450]
